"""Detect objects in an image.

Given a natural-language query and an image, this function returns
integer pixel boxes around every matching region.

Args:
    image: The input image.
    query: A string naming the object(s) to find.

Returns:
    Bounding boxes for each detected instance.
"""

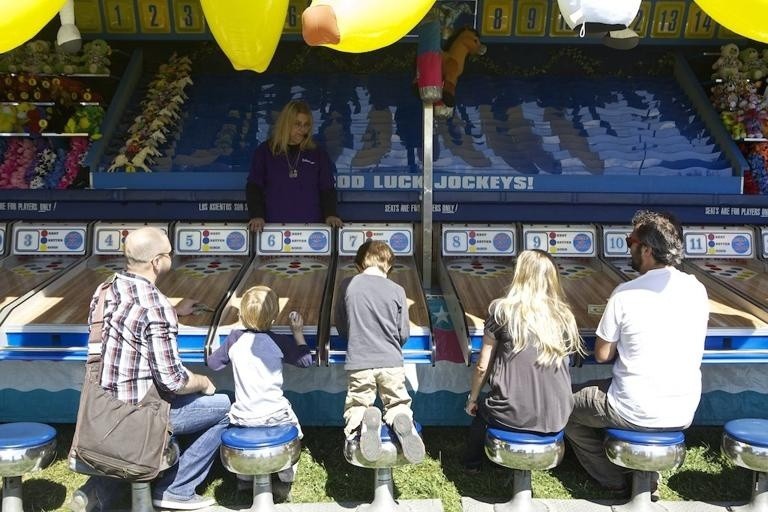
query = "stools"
[717,415,767,512]
[480,424,568,512]
[602,427,687,512]
[343,418,425,512]
[118,436,183,511]
[1,419,60,512]
[218,424,303,510]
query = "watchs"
[467,394,476,403]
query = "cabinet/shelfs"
[0,71,111,137]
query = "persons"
[564,208,711,495]
[341,238,425,466]
[463,249,582,476]
[206,283,314,491]
[85,227,237,510]
[244,98,345,233]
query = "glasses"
[626,237,642,247]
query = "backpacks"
[68,363,172,482]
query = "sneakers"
[393,414,426,465]
[71,486,100,510]
[152,488,215,510]
[358,407,383,462]
